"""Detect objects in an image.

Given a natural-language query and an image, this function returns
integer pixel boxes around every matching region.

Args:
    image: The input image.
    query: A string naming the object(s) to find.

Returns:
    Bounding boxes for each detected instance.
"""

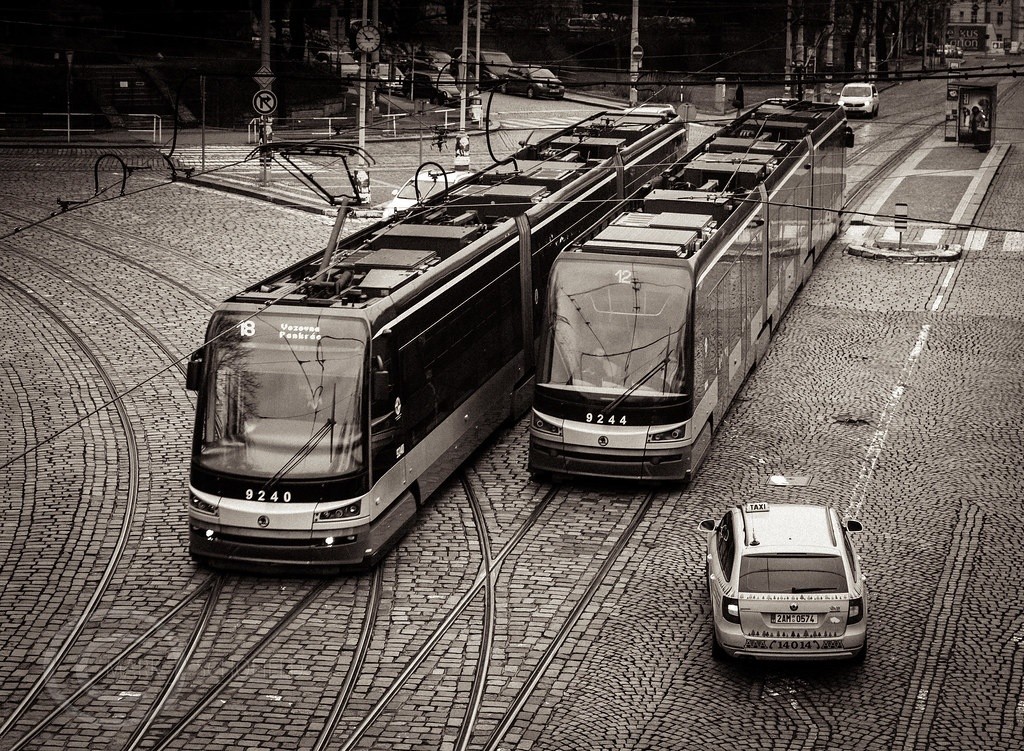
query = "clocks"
[354,24,382,52]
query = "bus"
[186,102,689,573]
[527,95,855,488]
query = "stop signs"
[632,46,643,61]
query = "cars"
[353,63,406,92]
[501,65,566,100]
[313,50,360,79]
[407,50,454,74]
[402,71,479,107]
[914,43,963,58]
[1003,38,1024,56]
[837,83,879,117]
[377,44,408,62]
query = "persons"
[968,106,985,149]
[735,83,744,117]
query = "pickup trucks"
[536,18,616,36]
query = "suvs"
[697,503,870,666]
[449,47,518,87]
